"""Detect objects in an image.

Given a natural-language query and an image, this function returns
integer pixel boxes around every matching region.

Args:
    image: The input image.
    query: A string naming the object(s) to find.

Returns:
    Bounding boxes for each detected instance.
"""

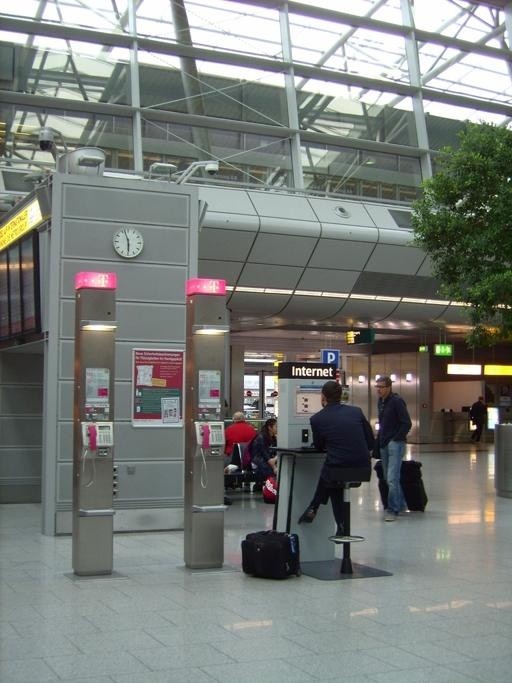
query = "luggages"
[373,461,428,512]
[241,453,302,579]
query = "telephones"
[195,421,227,448]
[80,421,115,449]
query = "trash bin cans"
[494,423,512,499]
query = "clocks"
[112,227,144,259]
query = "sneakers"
[385,510,397,521]
[303,505,317,523]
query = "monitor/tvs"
[295,391,324,418]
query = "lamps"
[145,162,177,182]
[77,156,103,176]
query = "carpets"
[300,558,393,581]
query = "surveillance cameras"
[204,164,219,175]
[38,129,54,151]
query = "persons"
[299,382,375,543]
[226,412,257,457]
[376,378,412,522]
[470,396,487,444]
[245,420,278,483]
[247,390,278,397]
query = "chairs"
[224,443,256,492]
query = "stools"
[328,463,371,574]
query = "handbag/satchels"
[243,470,266,482]
[371,434,381,459]
[243,448,251,464]
